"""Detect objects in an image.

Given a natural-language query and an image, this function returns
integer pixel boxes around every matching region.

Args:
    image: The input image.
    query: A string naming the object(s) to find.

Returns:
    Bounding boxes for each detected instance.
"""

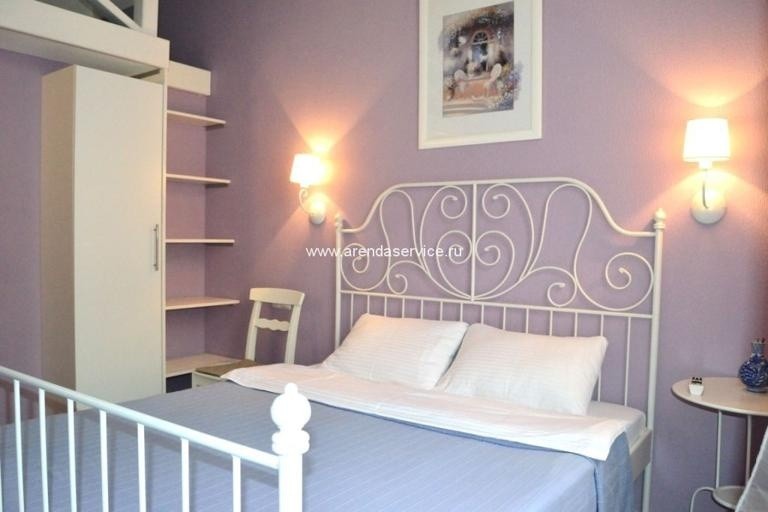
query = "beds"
[0,177,666,512]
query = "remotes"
[688,376,704,396]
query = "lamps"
[289,155,328,225]
[683,118,732,226]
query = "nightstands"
[671,377,768,512]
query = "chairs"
[191,287,305,387]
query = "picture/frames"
[418,0,542,150]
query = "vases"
[739,342,768,394]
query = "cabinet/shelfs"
[163,110,240,378]
[41,65,164,411]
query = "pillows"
[434,323,608,417]
[321,313,468,391]
